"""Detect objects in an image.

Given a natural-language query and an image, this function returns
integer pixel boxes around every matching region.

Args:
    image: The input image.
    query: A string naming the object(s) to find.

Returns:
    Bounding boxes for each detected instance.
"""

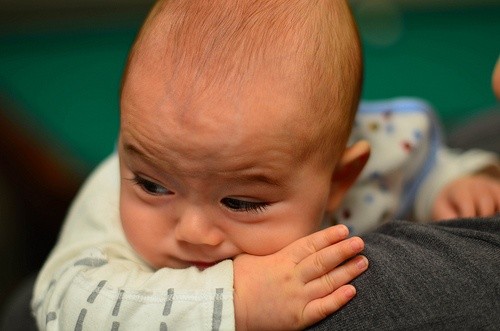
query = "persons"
[304,54,500,331]
[30,1,500,331]
